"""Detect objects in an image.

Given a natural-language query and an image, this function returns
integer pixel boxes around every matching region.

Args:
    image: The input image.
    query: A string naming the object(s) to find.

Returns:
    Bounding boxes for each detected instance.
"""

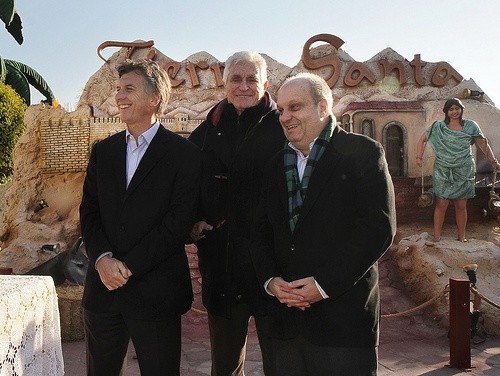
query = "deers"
[20,236,88,290]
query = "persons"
[415,97,500,244]
[251,71,395,376]
[79,57,202,376]
[188,50,284,376]
